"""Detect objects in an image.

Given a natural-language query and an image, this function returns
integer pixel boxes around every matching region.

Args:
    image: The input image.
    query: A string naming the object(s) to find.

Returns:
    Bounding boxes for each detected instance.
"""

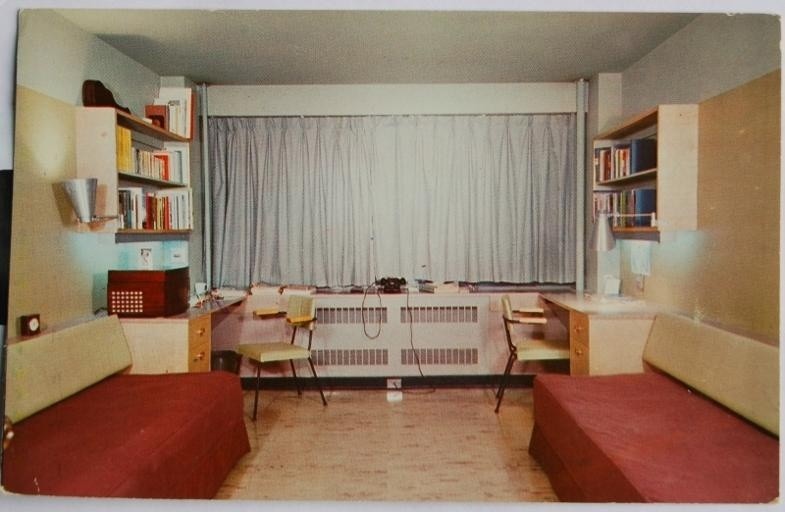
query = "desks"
[110,294,246,373]
[536,292,657,376]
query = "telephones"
[379,277,406,294]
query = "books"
[421,283,460,293]
[282,285,318,295]
[593,188,657,229]
[144,96,188,137]
[594,138,657,183]
[116,124,184,184]
[118,186,191,232]
[250,285,282,295]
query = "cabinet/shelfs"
[69,106,194,233]
[593,103,699,232]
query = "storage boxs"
[107,266,189,318]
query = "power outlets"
[386,379,401,388]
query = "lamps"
[62,179,117,223]
[589,210,656,252]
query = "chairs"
[234,294,328,421]
[494,294,570,413]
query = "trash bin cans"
[211,350,243,374]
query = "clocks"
[21,314,41,336]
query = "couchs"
[4,313,250,498]
[529,314,782,504]
